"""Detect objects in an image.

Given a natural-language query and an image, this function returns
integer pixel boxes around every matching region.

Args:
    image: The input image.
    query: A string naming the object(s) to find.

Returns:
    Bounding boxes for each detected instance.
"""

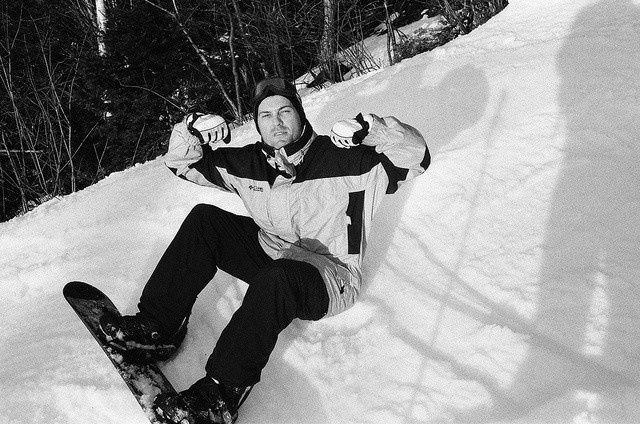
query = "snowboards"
[62,281,217,424]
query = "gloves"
[187,112,233,147]
[330,113,374,150]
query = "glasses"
[255,77,289,102]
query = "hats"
[254,80,307,136]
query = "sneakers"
[99,311,189,363]
[153,376,254,424]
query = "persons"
[100,77,430,423]
